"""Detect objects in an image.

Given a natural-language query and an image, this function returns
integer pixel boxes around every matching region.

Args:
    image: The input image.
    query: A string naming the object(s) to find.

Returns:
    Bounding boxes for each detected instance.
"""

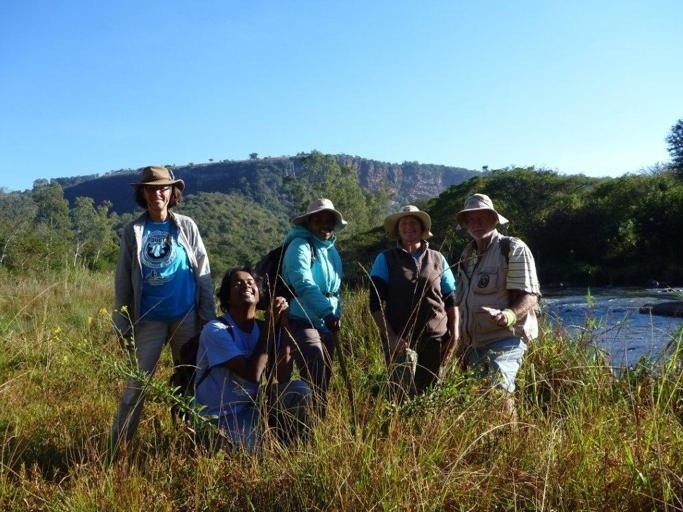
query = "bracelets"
[500,308,518,329]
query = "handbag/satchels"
[499,235,544,318]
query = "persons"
[452,194,543,431]
[99,166,217,471]
[276,196,348,420]
[367,204,460,405]
[189,267,315,467]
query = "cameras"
[259,285,298,311]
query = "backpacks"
[172,315,235,399]
[256,238,314,310]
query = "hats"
[384,205,433,241]
[456,194,509,225]
[130,167,184,194]
[293,198,348,231]
